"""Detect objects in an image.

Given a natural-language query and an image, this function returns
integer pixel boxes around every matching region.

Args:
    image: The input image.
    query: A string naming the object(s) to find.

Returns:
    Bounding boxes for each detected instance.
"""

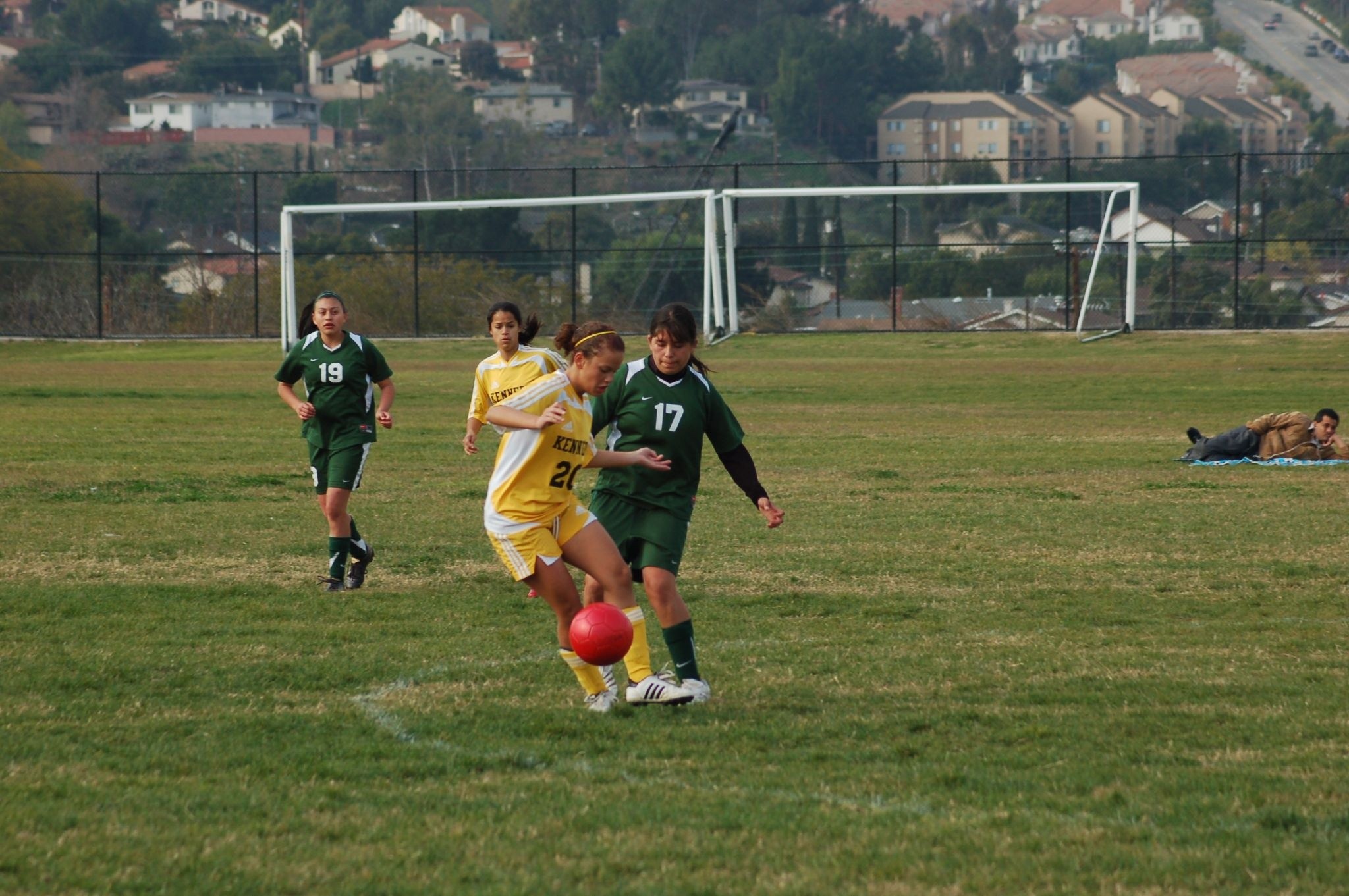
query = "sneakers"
[345,544,374,589]
[317,575,345,592]
[626,661,694,707]
[679,679,711,706]
[599,664,617,695]
[584,690,619,712]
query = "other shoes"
[1186,427,1202,444]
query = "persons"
[1185,407,1349,462]
[483,320,695,715]
[461,300,571,456]
[588,305,785,708]
[273,291,396,593]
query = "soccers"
[570,604,633,666]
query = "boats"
[1308,316,1338,328]
[1054,294,1064,307]
[952,295,963,304]
